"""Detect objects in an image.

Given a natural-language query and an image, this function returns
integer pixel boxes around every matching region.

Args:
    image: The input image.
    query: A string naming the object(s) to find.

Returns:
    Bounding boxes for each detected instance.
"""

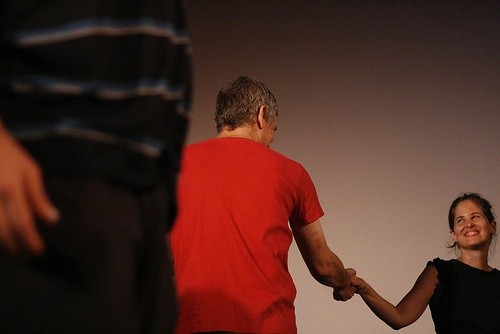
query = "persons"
[167,74,357,334]
[0,0,195,334]
[334,194,500,334]
